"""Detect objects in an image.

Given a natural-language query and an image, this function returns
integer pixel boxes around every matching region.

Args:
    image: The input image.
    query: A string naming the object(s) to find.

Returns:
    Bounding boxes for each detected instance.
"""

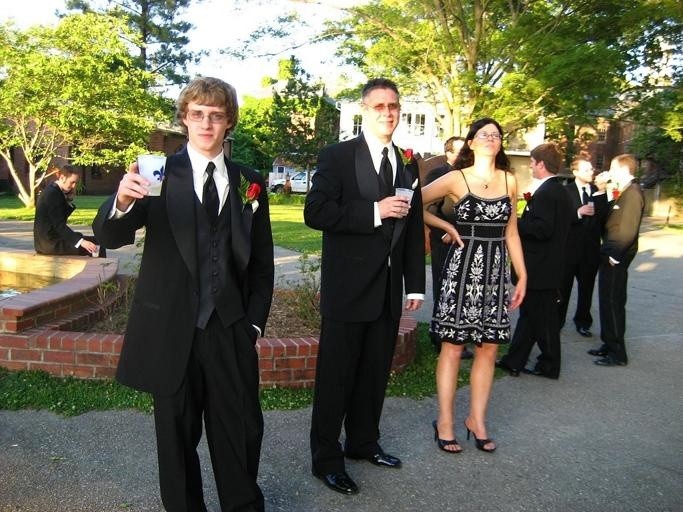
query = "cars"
[269,172,313,194]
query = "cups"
[587,201,594,207]
[91,244,101,258]
[136,154,167,199]
[394,187,414,207]
